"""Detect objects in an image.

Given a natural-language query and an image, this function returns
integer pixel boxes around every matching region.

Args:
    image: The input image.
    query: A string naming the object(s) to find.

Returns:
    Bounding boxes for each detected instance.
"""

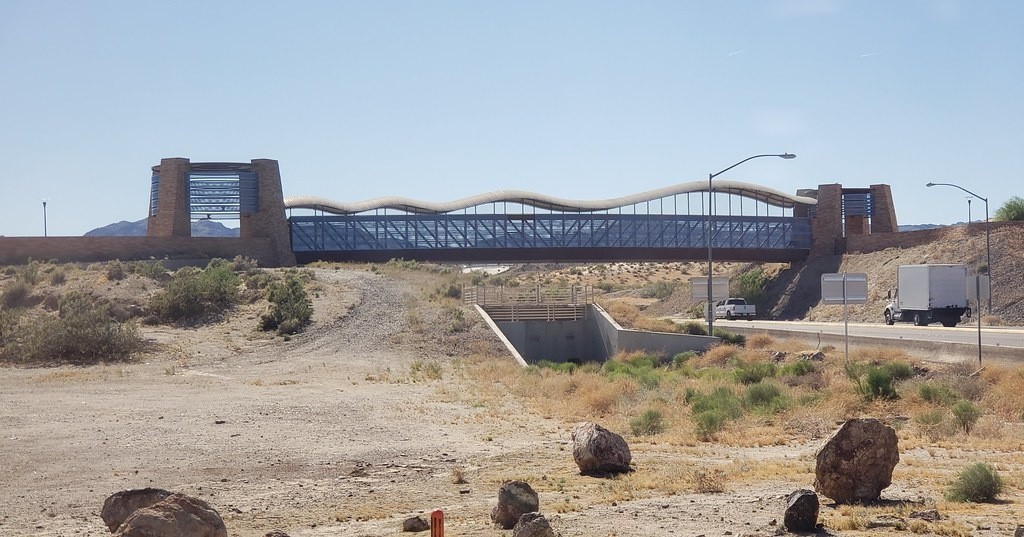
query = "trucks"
[884,264,971,327]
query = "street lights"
[41,198,48,236]
[926,183,993,314]
[708,153,798,338]
[965,196,973,224]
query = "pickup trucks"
[715,298,757,321]
